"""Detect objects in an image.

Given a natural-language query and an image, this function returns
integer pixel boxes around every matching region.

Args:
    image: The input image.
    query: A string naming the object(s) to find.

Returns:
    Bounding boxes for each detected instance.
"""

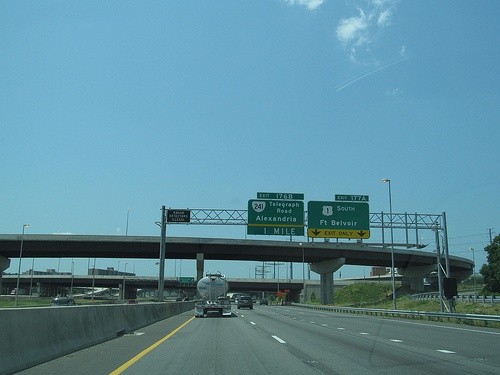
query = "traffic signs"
[306,200,371,239]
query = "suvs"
[237,296,254,309]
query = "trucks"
[193,272,234,317]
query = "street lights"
[381,177,397,309]
[469,248,477,292]
[122,263,129,300]
[15,224,31,306]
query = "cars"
[260,299,268,305]
[176,298,183,301]
[251,298,258,302]
[51,296,76,306]
[185,298,189,301]
[230,298,239,303]
